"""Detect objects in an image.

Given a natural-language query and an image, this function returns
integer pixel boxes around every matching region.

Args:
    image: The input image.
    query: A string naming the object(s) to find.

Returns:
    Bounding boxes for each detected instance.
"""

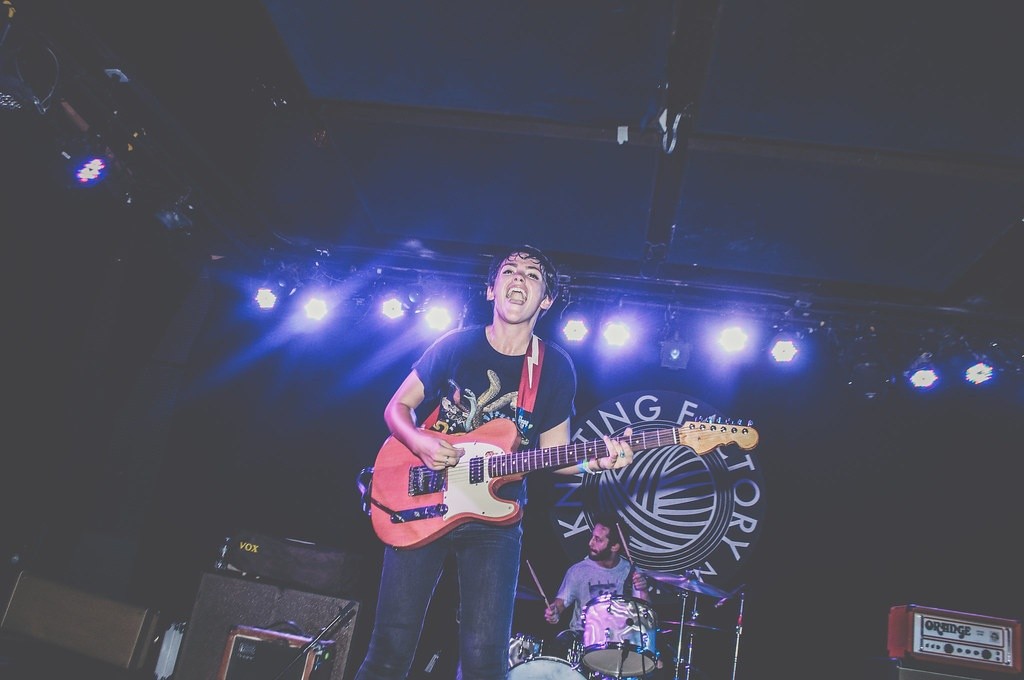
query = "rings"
[611,459,616,463]
[445,456,450,464]
[639,581,642,585]
[620,451,625,458]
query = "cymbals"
[638,565,737,604]
[662,615,722,634]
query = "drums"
[508,632,544,668]
[582,592,660,675]
[506,656,583,680]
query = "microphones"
[713,584,744,608]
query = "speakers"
[218,619,336,680]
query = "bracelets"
[595,459,604,471]
[582,461,596,476]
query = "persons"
[351,241,633,680]
[543,512,650,660]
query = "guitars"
[365,412,760,551]
